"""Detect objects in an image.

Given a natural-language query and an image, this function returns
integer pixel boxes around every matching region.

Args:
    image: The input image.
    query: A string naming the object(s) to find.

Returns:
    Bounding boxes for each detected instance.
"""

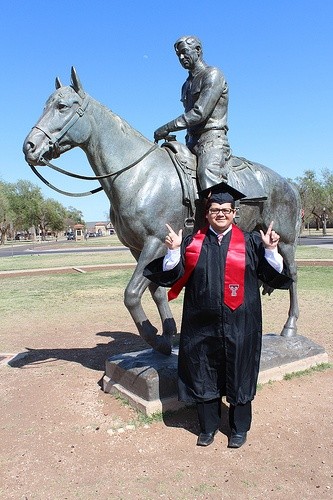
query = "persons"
[163,183,293,448]
[154,36,231,191]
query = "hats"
[197,182,247,205]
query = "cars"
[89,232,96,237]
[67,234,75,240]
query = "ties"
[217,234,223,246]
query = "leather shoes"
[197,429,219,446]
[228,432,246,448]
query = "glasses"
[207,208,233,214]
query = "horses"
[23,66,302,360]
